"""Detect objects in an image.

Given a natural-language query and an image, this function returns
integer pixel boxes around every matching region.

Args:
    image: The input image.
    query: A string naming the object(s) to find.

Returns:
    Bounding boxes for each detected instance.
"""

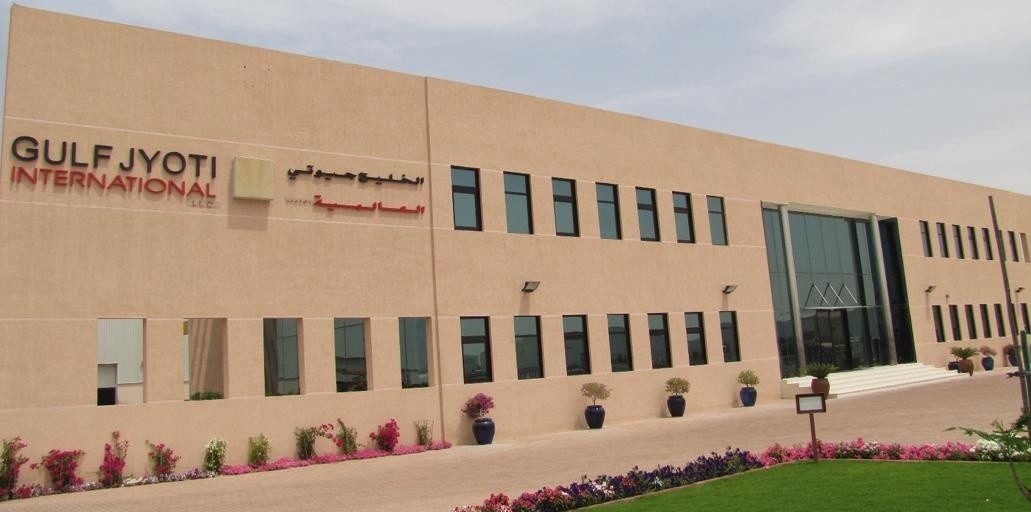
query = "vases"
[470,417,497,445]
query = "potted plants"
[577,380,613,428]
[948,344,1022,375]
[736,368,761,408]
[664,377,691,418]
[799,362,841,400]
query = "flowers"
[414,417,431,447]
[40,446,86,488]
[97,430,131,485]
[337,417,363,457]
[1,435,31,494]
[203,434,229,477]
[298,420,343,455]
[143,440,182,482]
[459,390,499,421]
[249,434,271,466]
[369,420,402,452]
